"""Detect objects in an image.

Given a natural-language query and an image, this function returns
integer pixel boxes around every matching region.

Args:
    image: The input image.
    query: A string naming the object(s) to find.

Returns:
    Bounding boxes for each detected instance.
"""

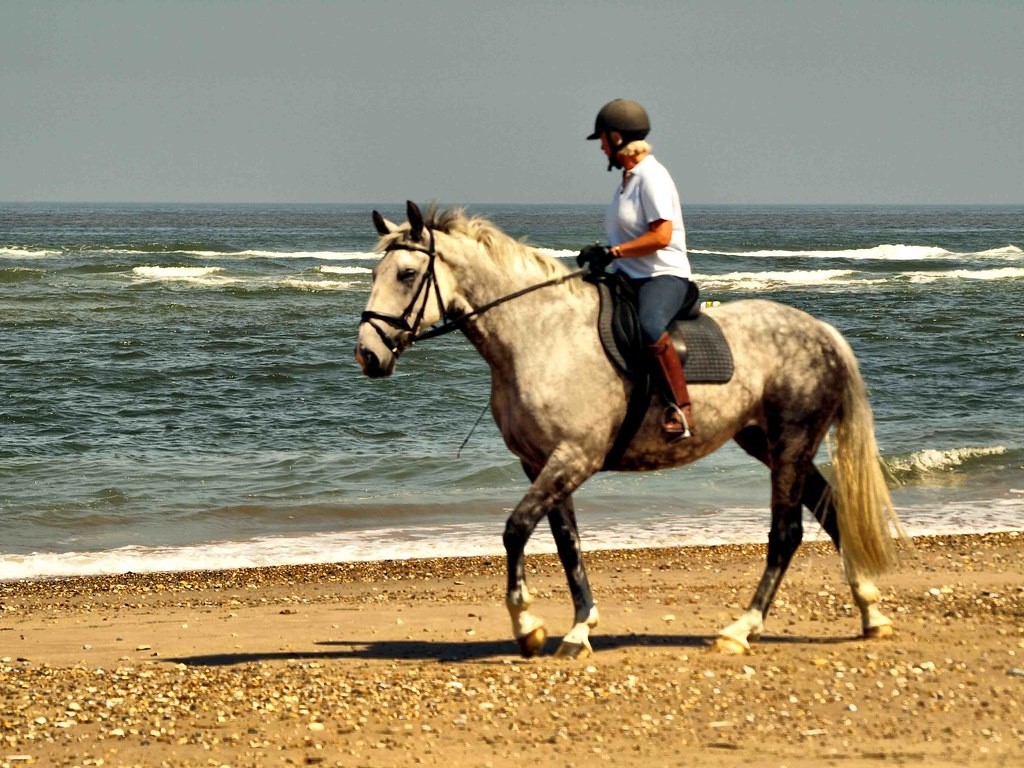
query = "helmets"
[585,98,652,140]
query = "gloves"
[588,245,616,278]
[575,240,604,269]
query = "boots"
[645,332,696,435]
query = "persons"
[576,98,696,434]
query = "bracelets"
[615,245,623,257]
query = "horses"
[354,199,916,659]
